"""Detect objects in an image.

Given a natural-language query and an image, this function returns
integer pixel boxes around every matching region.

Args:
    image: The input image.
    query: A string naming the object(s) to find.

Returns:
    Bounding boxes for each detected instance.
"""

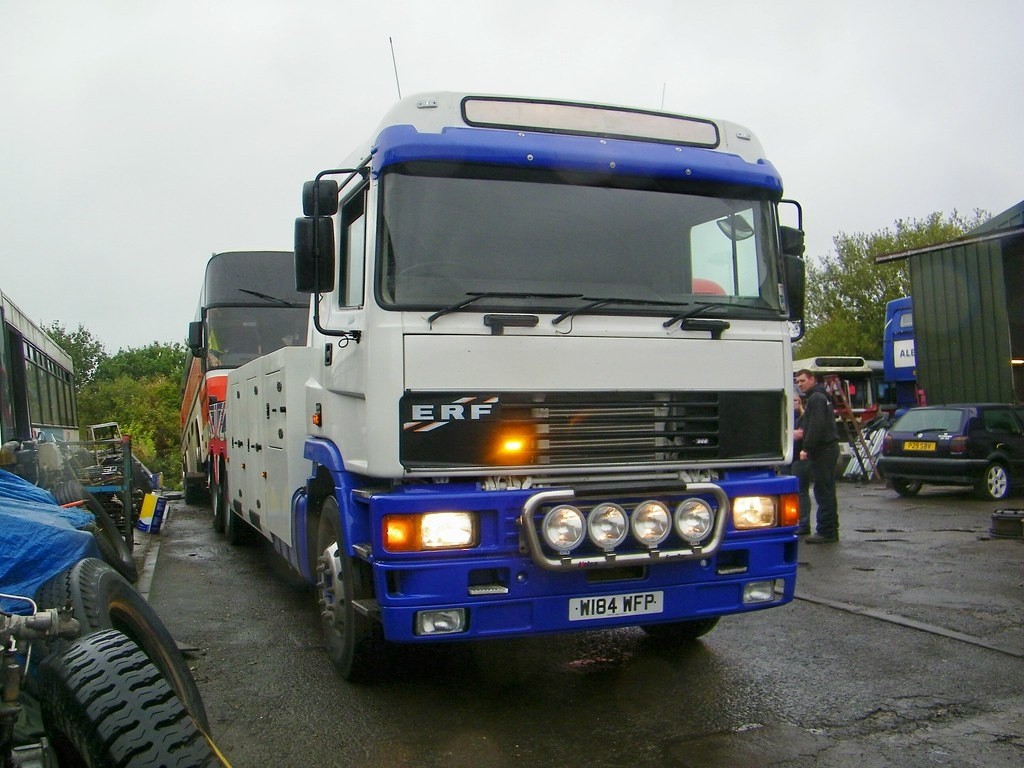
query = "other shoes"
[794,528,811,534]
[805,535,839,544]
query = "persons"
[790,369,839,544]
[794,390,802,462]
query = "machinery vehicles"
[216,91,810,684]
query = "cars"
[876,403,1024,501]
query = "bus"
[178,249,310,506]
[792,355,889,479]
